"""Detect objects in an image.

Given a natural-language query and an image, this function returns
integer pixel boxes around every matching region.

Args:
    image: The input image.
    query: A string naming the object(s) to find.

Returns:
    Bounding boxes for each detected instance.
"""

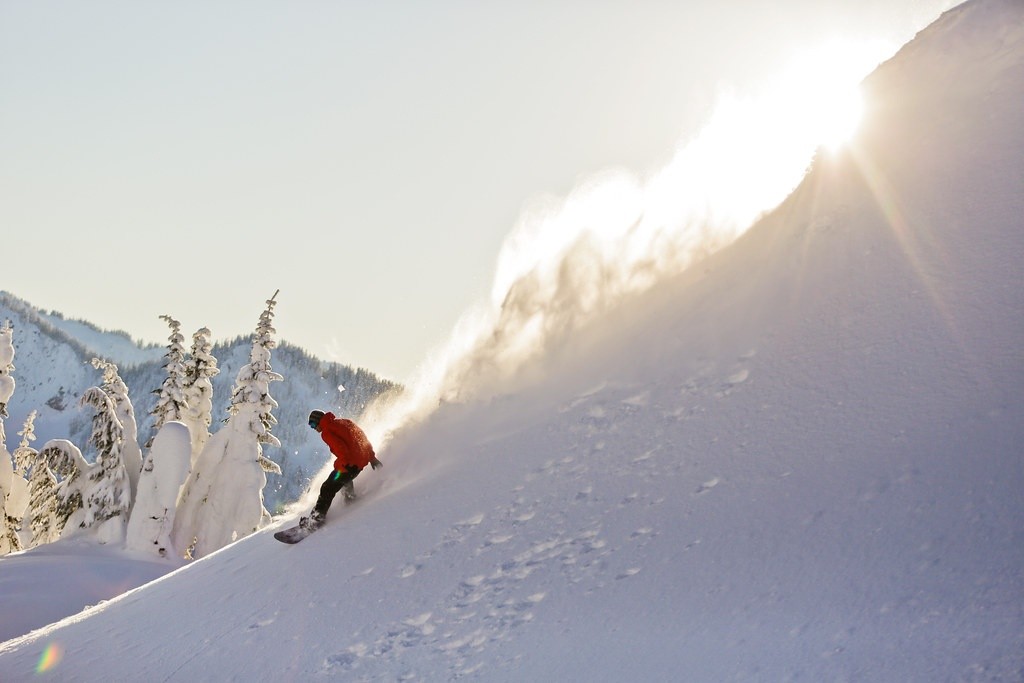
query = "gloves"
[344,465,357,473]
[372,458,383,470]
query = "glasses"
[311,424,318,428]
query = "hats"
[308,409,325,425]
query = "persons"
[298,409,383,531]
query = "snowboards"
[274,519,326,544]
